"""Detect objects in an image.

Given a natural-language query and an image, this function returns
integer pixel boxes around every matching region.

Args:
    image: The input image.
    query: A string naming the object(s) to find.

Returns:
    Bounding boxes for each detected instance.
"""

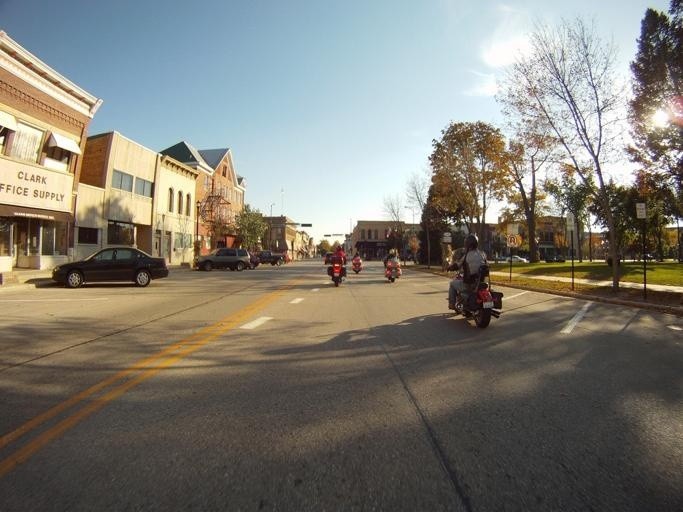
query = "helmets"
[464,234,478,249]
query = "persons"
[448,234,486,309]
[352,251,361,262]
[329,245,347,265]
[384,247,400,266]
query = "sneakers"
[449,303,456,310]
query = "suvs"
[196,248,250,271]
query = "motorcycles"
[384,261,402,282]
[327,256,346,287]
[352,258,363,274]
[447,264,503,328]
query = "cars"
[52,246,169,287]
[641,253,654,260]
[545,255,578,263]
[487,253,530,263]
[249,253,260,267]
[324,252,334,264]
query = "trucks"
[256,250,284,266]
